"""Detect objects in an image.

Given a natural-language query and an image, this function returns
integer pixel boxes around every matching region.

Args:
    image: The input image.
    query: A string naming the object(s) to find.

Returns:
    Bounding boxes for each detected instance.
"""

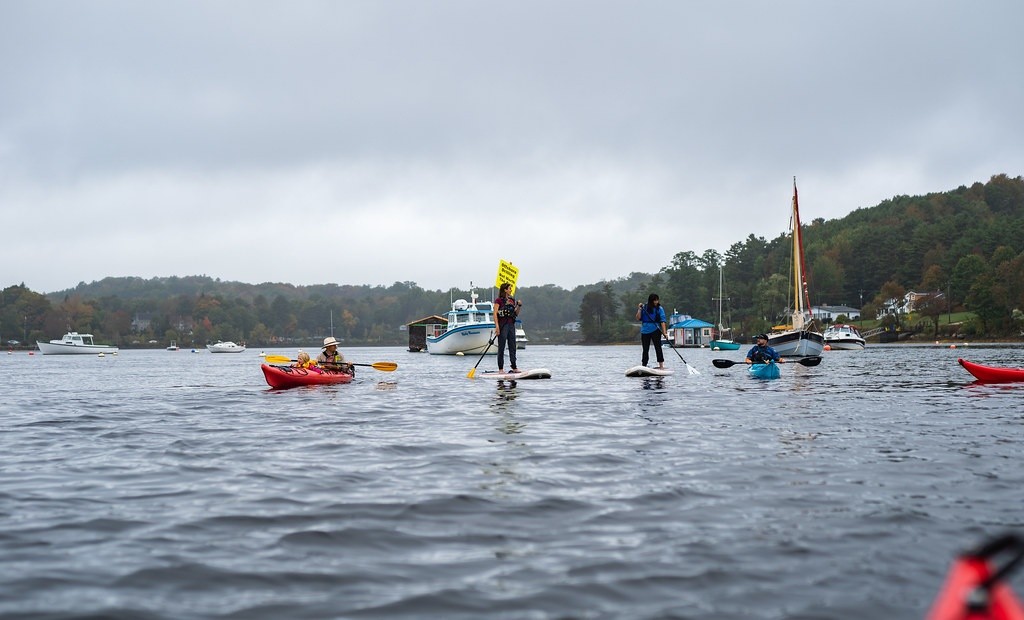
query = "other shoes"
[499,369,505,374]
[511,368,521,373]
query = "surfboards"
[478,368,553,380]
[625,365,674,378]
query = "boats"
[513,319,529,349]
[206,339,246,353]
[167,339,177,350]
[425,279,507,356]
[660,329,675,348]
[36,332,119,355]
[823,323,866,350]
[958,357,1024,382]
[260,362,353,389]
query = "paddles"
[712,357,822,368]
[265,355,398,372]
[466,301,519,378]
[641,305,700,375]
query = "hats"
[322,337,340,349]
[755,334,768,342]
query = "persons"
[746,334,783,366]
[636,294,667,370]
[493,283,522,374]
[316,336,353,372]
[292,353,318,369]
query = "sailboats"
[709,263,741,350]
[765,175,824,356]
